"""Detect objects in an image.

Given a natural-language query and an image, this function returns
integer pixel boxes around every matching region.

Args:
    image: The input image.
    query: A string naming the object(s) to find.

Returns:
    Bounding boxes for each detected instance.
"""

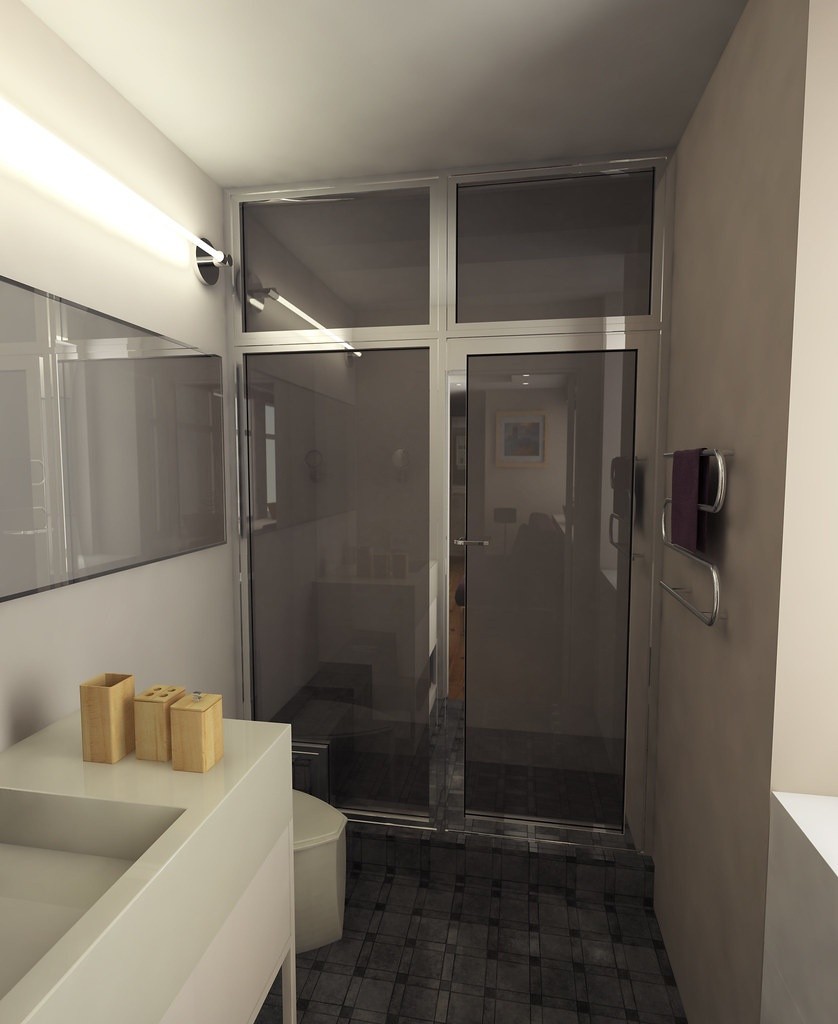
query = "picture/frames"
[495,411,549,470]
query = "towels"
[670,446,712,554]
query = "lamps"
[248,287,362,358]
[0,96,232,285]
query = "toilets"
[292,788,347,955]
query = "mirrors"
[0,277,226,607]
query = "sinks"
[0,783,189,1008]
